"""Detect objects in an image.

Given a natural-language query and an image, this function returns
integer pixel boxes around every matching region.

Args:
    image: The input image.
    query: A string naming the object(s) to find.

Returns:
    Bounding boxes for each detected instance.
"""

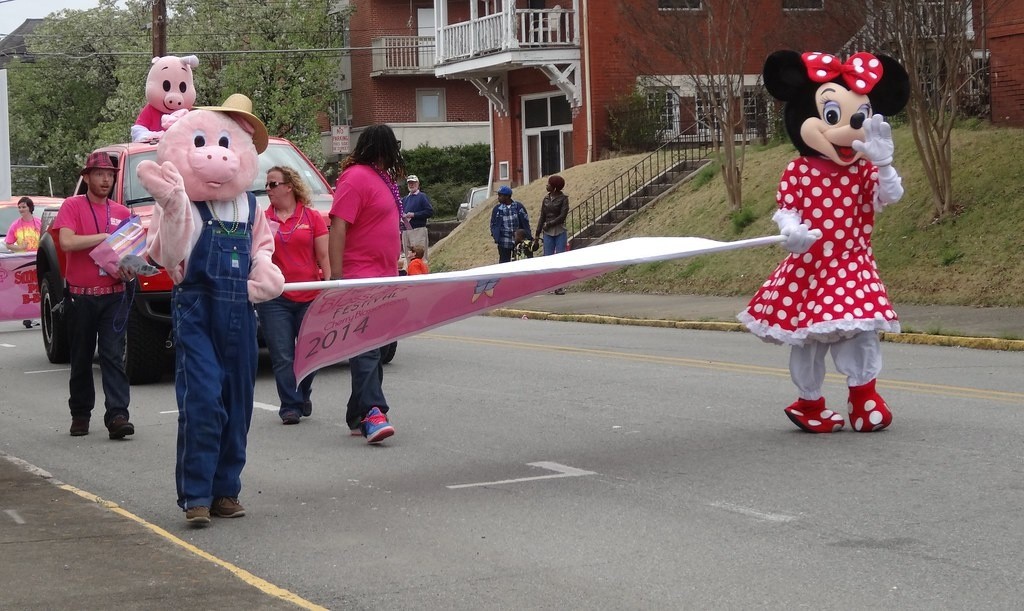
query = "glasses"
[265,181,289,188]
[395,140,401,149]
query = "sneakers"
[186,507,210,523]
[352,406,394,443]
[212,498,245,518]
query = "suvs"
[35,138,398,386]
[0,195,68,255]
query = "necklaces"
[277,207,305,243]
[364,161,404,217]
[210,199,239,234]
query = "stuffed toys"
[130,56,201,141]
[759,50,910,434]
[136,94,286,522]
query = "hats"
[407,245,425,258]
[548,176,566,190]
[407,175,419,182]
[494,186,513,195]
[80,152,121,175]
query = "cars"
[457,185,489,222]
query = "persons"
[328,124,409,442]
[491,186,532,263]
[51,153,134,439]
[253,166,332,426]
[396,175,433,276]
[511,229,539,262]
[534,175,569,256]
[5,197,42,328]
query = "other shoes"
[108,416,135,437]
[68,417,92,436]
[281,403,312,424]
[555,289,566,294]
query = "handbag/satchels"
[88,214,148,281]
